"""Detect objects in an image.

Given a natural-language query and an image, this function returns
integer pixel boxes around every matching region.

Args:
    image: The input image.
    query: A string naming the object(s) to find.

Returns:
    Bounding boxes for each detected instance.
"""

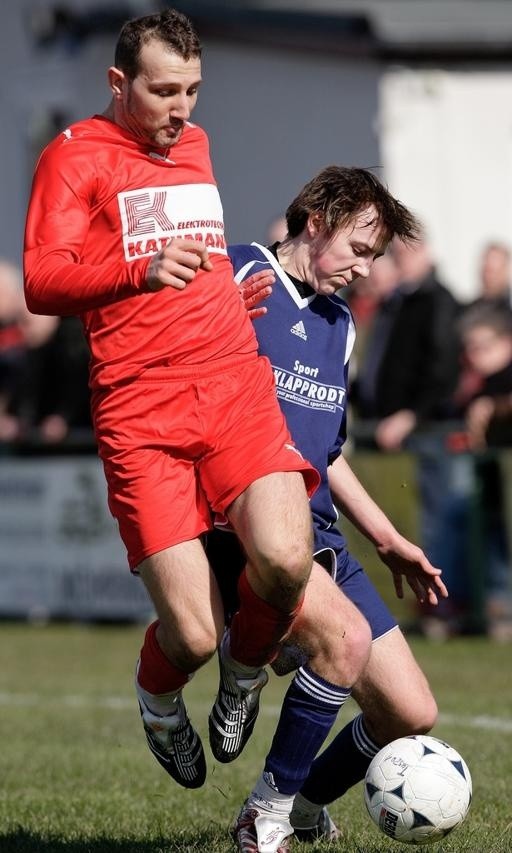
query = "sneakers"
[136,661,206,788]
[235,795,343,853]
[209,629,269,762]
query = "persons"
[0,257,103,455]
[21,8,324,788]
[229,165,450,850]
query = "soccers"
[364,734,473,843]
